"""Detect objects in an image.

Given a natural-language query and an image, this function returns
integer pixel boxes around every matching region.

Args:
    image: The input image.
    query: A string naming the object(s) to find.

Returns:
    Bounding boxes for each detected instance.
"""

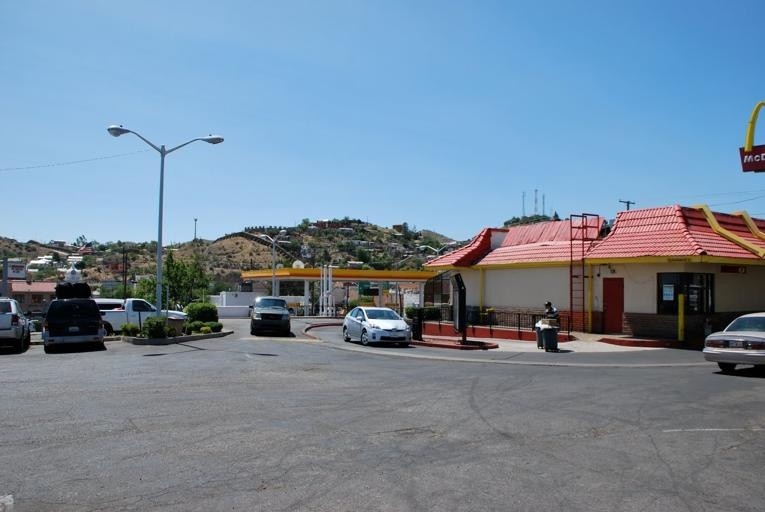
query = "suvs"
[0,297,31,352]
[247,295,294,336]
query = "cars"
[703,310,765,372]
[343,306,412,347]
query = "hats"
[543,301,552,306]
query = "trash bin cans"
[304,305,310,316]
[248,305,253,316]
[534,326,559,352]
[410,317,423,340]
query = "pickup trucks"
[100,297,190,336]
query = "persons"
[543,301,560,335]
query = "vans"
[90,298,124,310]
[41,298,106,353]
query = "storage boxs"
[540,318,558,326]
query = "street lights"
[255,229,292,295]
[106,126,226,322]
[419,241,457,255]
